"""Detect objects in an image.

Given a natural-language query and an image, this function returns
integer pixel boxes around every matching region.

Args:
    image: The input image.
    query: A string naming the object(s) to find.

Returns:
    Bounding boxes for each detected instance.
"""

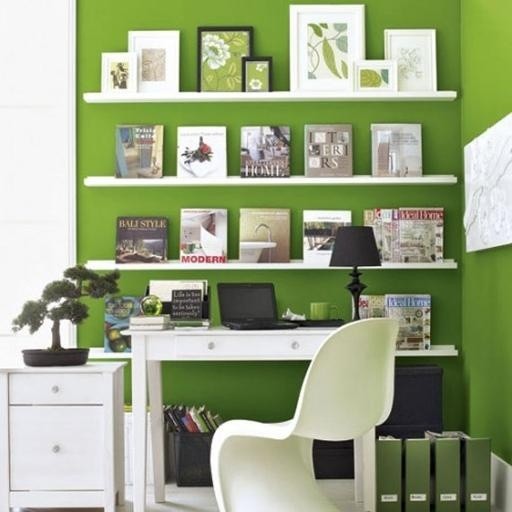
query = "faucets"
[254,224,273,263]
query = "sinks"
[240,242,278,263]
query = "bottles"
[389,152,398,175]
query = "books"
[351,295,384,320]
[127,324,170,332]
[370,123,423,178]
[163,404,223,435]
[304,123,353,178]
[364,208,445,264]
[114,124,163,178]
[239,125,292,178]
[238,207,291,263]
[128,313,170,324]
[176,125,228,178]
[103,295,141,353]
[179,208,228,264]
[384,294,431,350]
[114,215,167,265]
[303,209,353,263]
[173,320,210,330]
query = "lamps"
[328,226,383,327]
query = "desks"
[116,320,391,510]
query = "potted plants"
[12,263,126,368]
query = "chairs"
[208,316,404,509]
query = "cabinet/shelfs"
[82,84,463,276]
[1,356,130,511]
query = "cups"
[309,301,339,321]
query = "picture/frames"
[241,55,273,92]
[100,51,139,97]
[288,4,367,93]
[382,27,438,93]
[127,29,182,92]
[196,25,253,92]
[352,59,400,93]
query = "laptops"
[217,282,298,330]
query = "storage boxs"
[373,361,446,437]
[168,424,224,488]
